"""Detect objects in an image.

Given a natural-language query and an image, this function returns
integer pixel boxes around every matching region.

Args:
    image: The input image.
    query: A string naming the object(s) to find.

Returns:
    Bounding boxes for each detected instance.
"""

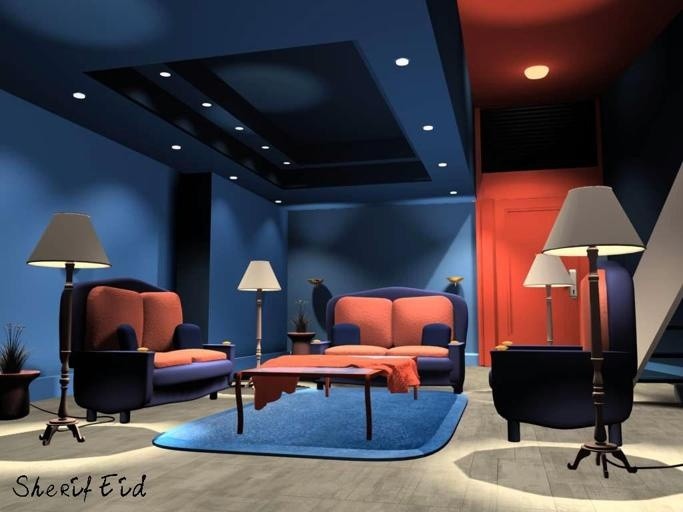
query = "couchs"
[311,286,470,393]
[54,276,235,426]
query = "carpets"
[150,382,469,461]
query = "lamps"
[23,212,114,448]
[307,278,323,288]
[234,258,283,389]
[521,253,575,346]
[446,277,464,291]
[540,183,647,480]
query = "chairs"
[486,259,641,448]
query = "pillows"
[84,285,144,351]
[173,321,204,346]
[140,291,185,353]
[112,323,136,349]
[334,294,393,351]
[331,322,360,347]
[422,322,449,348]
[388,294,455,347]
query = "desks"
[287,332,316,354]
[230,353,419,440]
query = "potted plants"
[290,296,311,332]
[0,319,42,421]
